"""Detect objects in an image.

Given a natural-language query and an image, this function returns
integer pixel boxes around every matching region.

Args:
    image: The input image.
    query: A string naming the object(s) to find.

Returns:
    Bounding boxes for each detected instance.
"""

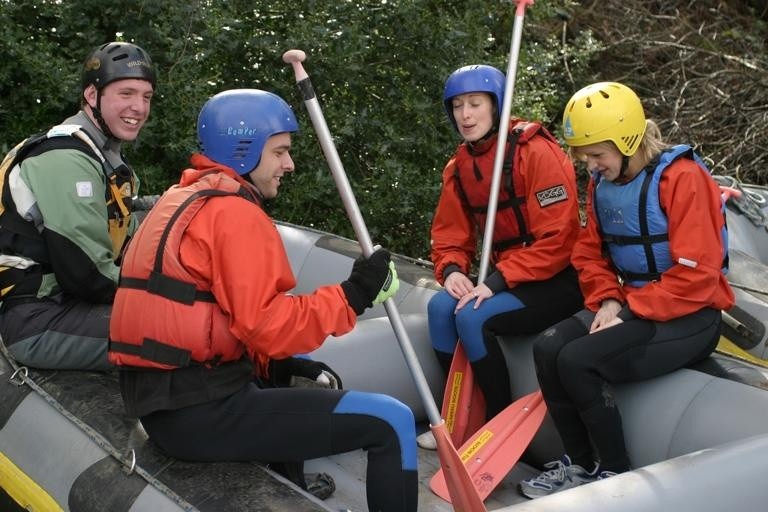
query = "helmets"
[196,88,299,176]
[561,81,648,157]
[443,64,506,133]
[81,42,157,107]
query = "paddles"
[440,1,535,453]
[282,49,490,512]
[430,184,743,503]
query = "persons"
[417,63,579,449]
[517,83,735,498]
[107,88,418,512]
[0,40,156,373]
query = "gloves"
[339,245,400,316]
[294,358,342,390]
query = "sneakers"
[517,453,602,499]
[416,429,437,450]
[303,472,335,500]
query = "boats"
[0,179,762,509]
